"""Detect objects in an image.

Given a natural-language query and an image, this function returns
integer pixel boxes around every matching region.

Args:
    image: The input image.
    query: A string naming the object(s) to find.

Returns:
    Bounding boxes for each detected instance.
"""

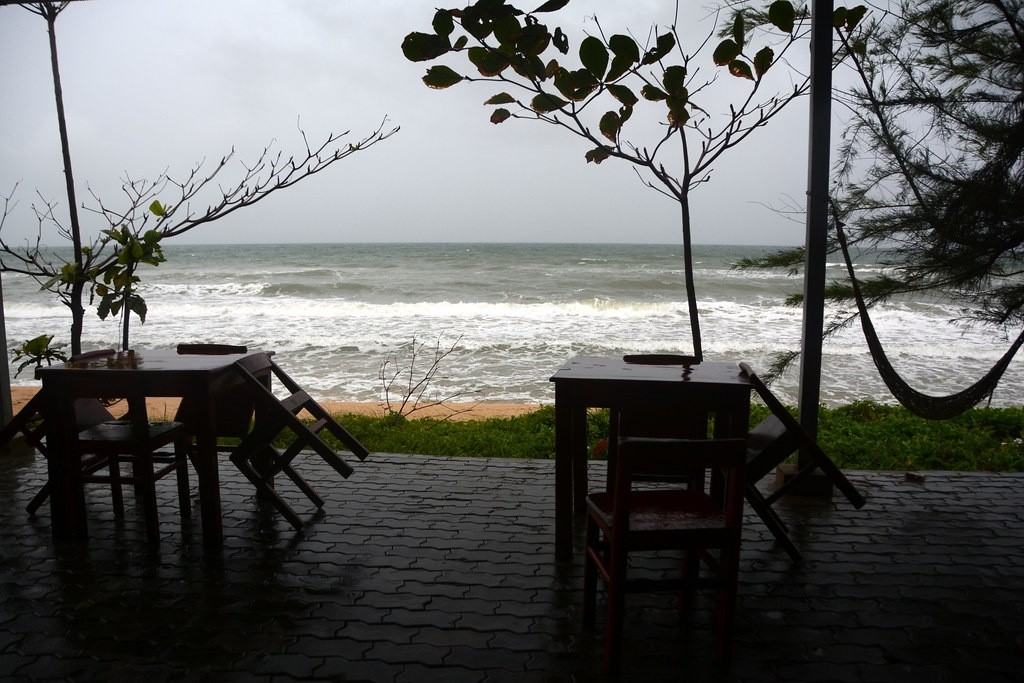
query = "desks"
[35,350,276,572]
[547,365,754,562]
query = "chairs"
[0,343,370,572]
[583,353,871,662]
